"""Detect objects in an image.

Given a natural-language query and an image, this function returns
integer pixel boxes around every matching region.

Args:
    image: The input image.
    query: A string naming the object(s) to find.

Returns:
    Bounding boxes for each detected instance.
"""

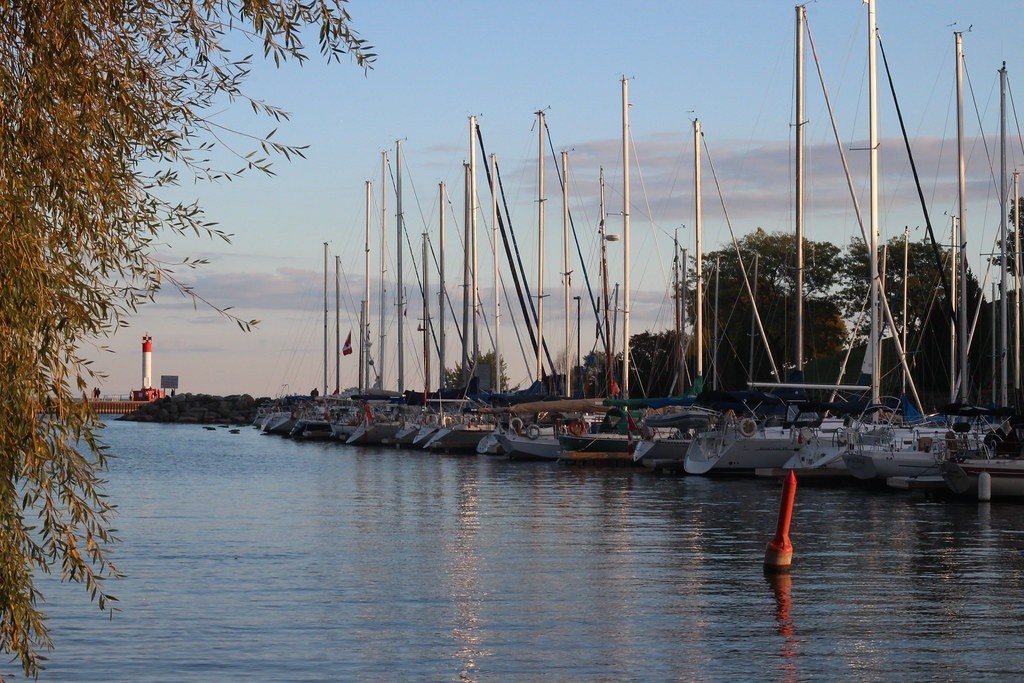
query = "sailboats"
[253,0,1023,499]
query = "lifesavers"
[570,419,585,437]
[526,424,540,440]
[511,418,522,431]
[258,408,265,415]
[426,413,438,427]
[740,418,756,437]
